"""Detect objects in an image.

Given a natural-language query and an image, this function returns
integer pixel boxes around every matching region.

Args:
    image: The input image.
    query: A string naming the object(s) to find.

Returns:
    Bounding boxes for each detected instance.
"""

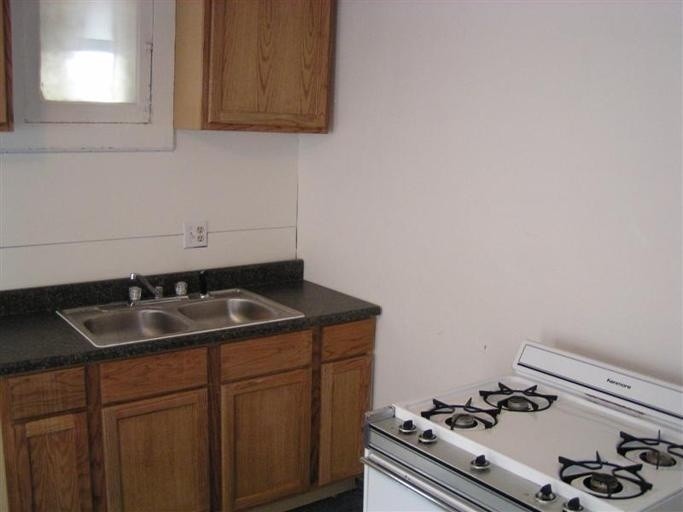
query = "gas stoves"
[365,375,683,512]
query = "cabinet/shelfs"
[172,0,338,135]
[0,316,378,512]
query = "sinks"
[54,301,192,348]
[177,288,306,331]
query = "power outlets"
[182,220,210,251]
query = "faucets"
[130,272,164,300]
